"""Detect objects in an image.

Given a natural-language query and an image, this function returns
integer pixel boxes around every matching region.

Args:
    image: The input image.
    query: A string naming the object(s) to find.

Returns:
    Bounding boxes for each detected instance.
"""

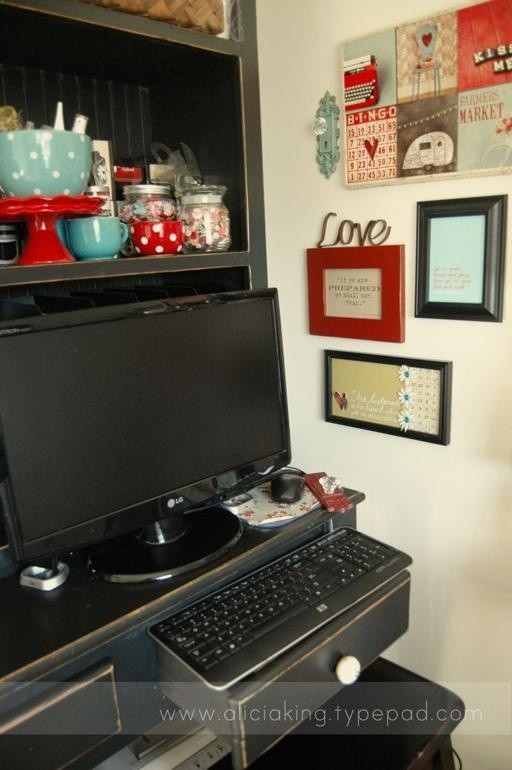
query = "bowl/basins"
[0,131,92,201]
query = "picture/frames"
[303,191,511,448]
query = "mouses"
[271,473,305,504]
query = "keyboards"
[145,525,413,692]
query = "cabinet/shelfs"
[3,2,258,284]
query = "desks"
[3,468,416,645]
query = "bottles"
[122,184,231,256]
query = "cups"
[67,217,130,264]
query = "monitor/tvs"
[1,287,292,583]
